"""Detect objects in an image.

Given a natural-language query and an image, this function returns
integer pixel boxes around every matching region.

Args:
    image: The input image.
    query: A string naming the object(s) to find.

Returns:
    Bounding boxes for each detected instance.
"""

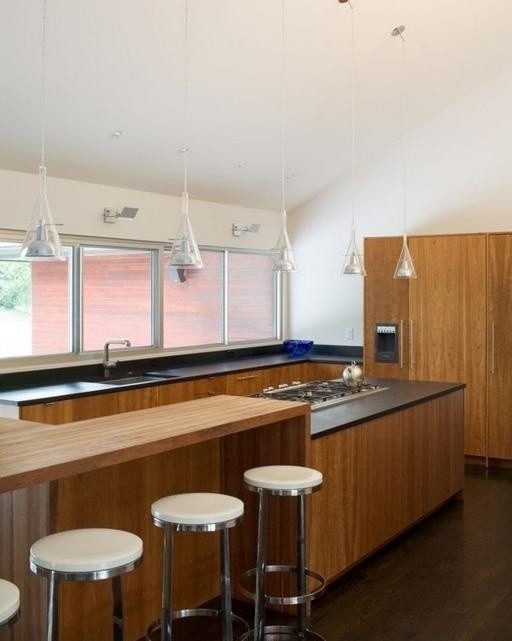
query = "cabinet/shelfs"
[360,235,410,381]
[408,230,488,475]
[0,379,469,620]
[484,230,512,481]
[0,355,361,421]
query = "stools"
[236,461,328,640]
[144,490,252,639]
[0,577,24,639]
[27,526,145,639]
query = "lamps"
[337,0,368,276]
[269,0,299,273]
[232,222,261,237]
[17,0,70,264]
[163,1,205,272]
[389,23,422,279]
[103,204,139,223]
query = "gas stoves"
[253,379,389,413]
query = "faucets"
[103,338,131,378]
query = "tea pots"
[342,361,364,389]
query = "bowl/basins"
[283,340,314,358]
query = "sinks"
[80,370,181,387]
